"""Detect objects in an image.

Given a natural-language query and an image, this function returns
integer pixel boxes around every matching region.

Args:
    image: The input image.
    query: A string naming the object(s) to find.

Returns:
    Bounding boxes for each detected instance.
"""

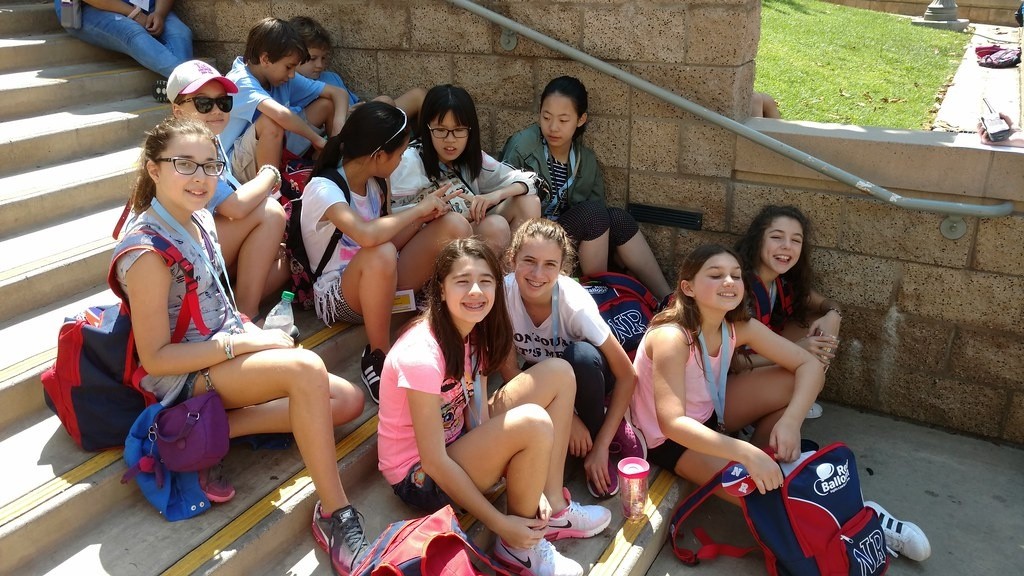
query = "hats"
[166,59,238,103]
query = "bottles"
[263,290,295,337]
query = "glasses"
[154,158,225,176]
[428,124,470,138]
[179,96,233,114]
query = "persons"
[721,203,842,442]
[53,0,193,103]
[113,116,373,576]
[625,244,931,561]
[752,93,781,119]
[977,112,1024,147]
[378,236,614,576]
[166,16,675,404]
[501,217,648,500]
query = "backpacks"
[39,232,211,452]
[575,273,665,363]
[670,438,890,576]
[273,159,388,312]
[352,504,536,576]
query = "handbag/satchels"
[153,391,230,472]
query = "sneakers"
[361,344,386,404]
[312,500,370,576]
[493,534,584,576]
[543,487,612,540]
[588,460,620,499]
[864,500,933,561]
[200,462,235,503]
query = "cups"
[813,329,840,368]
[616,457,651,520]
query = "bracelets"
[411,222,424,232]
[231,333,236,359]
[826,308,842,321]
[223,333,233,360]
[258,165,281,191]
[127,5,142,19]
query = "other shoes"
[806,404,823,419]
[152,80,170,102]
